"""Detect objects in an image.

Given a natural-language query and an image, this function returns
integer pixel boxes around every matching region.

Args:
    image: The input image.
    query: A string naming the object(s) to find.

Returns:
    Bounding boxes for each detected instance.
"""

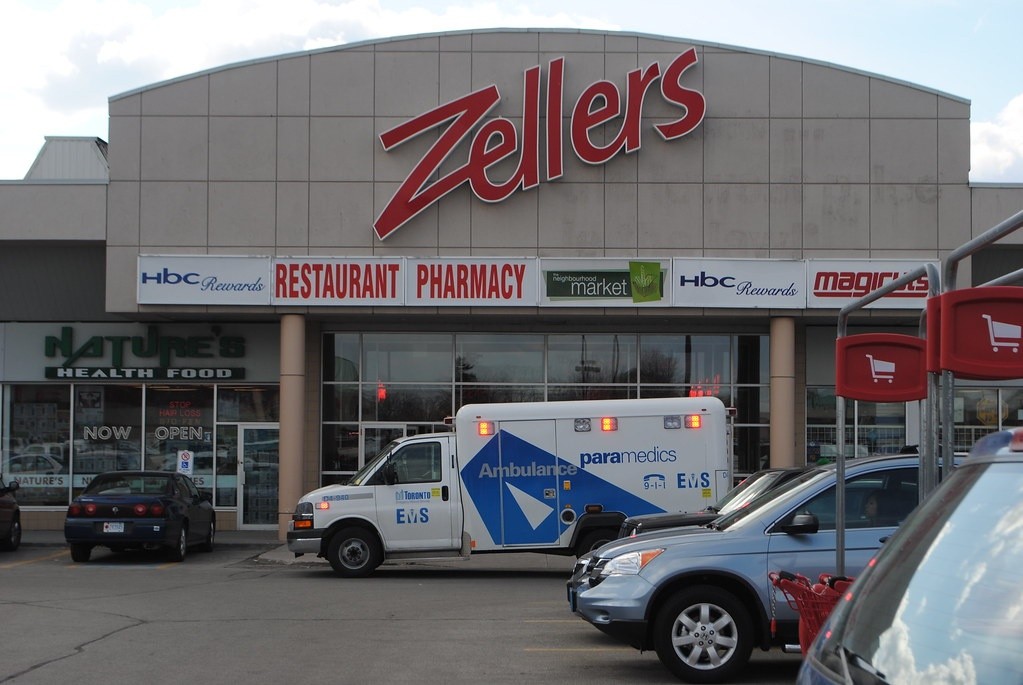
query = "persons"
[864,489,895,519]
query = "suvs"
[567,444,968,685]
[796,427,1023,685]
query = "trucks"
[287,397,737,577]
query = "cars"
[64,470,216,562]
[0,478,21,551]
